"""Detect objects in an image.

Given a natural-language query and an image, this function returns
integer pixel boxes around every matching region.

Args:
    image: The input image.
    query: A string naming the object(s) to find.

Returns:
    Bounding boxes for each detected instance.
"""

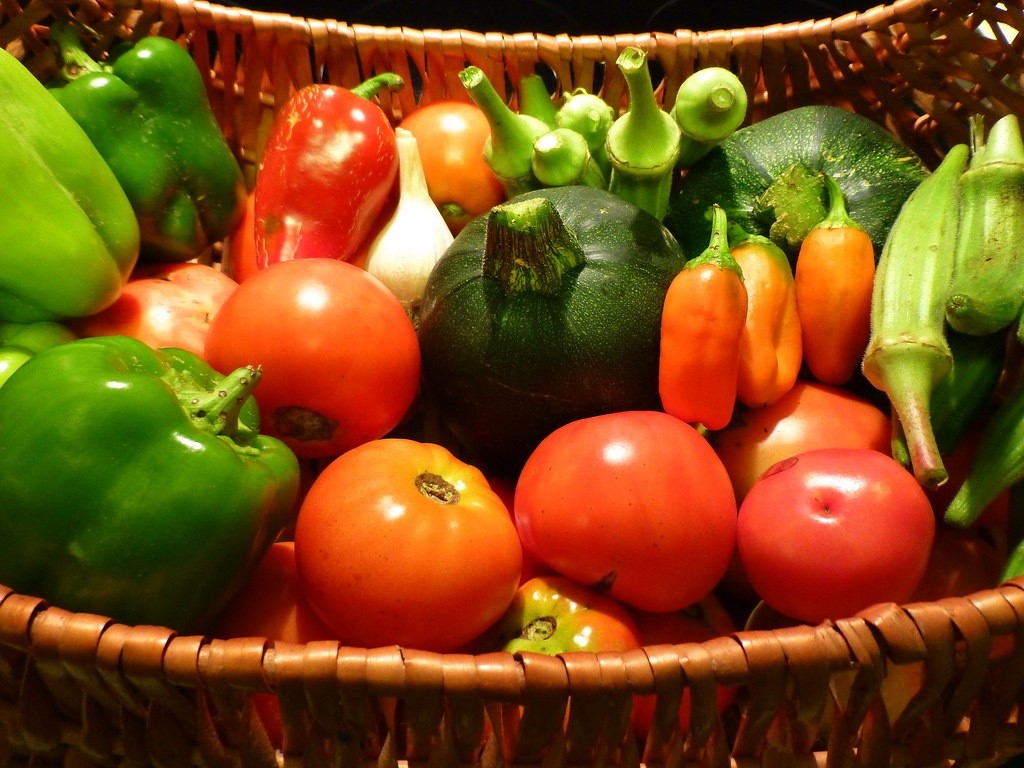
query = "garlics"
[363,126,455,319]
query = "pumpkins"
[668,104,936,259]
[410,184,685,467]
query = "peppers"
[0,21,301,636]
[659,168,877,433]
[221,71,408,261]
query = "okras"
[459,47,752,220]
[859,111,1024,592]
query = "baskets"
[0,0,1024,768]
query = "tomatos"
[80,260,934,768]
[383,99,508,224]
